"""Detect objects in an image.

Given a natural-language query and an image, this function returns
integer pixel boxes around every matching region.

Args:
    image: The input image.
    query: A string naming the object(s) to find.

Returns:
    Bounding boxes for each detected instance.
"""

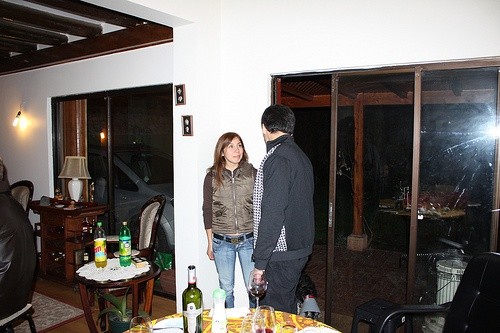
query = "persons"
[252,104,315,314]
[0,181,36,320]
[202,132,257,309]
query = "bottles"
[397,188,404,211]
[119,221,132,267]
[211,290,227,333]
[94,222,107,268]
[182,266,204,333]
[80,216,96,242]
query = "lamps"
[57,155,92,202]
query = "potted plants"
[96,284,132,333]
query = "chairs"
[89,194,167,317]
[374,250,500,333]
[9,179,34,216]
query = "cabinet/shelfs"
[31,201,110,282]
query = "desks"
[120,307,340,333]
[75,256,162,333]
[384,207,464,268]
[105,234,139,245]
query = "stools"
[0,303,37,333]
[352,298,407,333]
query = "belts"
[214,233,253,244]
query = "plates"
[153,317,184,333]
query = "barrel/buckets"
[437,260,468,326]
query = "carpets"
[5,289,84,333]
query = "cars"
[87,143,174,268]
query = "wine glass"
[248,270,267,310]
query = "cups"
[241,315,265,333]
[252,305,275,333]
[130,315,152,333]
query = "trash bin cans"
[436,260,470,305]
